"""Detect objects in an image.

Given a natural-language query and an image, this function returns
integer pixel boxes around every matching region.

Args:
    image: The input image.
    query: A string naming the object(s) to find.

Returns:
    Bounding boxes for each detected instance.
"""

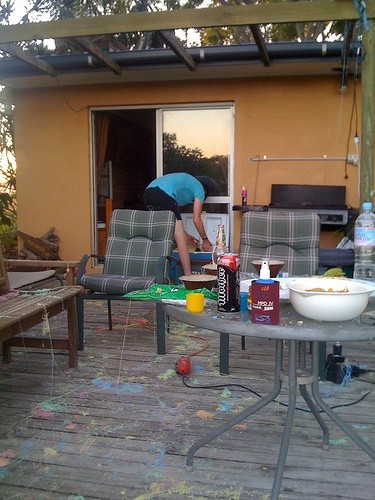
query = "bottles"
[241,186,247,206]
[333,340,343,356]
[250,259,280,324]
[353,202,375,297]
[212,224,227,264]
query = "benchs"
[0,260,83,367]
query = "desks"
[166,304,375,500]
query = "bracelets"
[202,237,208,241]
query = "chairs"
[218,210,325,381]
[76,209,175,354]
[17,232,59,260]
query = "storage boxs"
[168,213,229,284]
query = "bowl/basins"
[286,277,375,321]
[178,274,217,291]
[203,264,218,289]
[251,258,285,278]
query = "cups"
[186,292,204,313]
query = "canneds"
[217,253,241,312]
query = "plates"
[241,278,289,300]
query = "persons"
[143,172,212,275]
